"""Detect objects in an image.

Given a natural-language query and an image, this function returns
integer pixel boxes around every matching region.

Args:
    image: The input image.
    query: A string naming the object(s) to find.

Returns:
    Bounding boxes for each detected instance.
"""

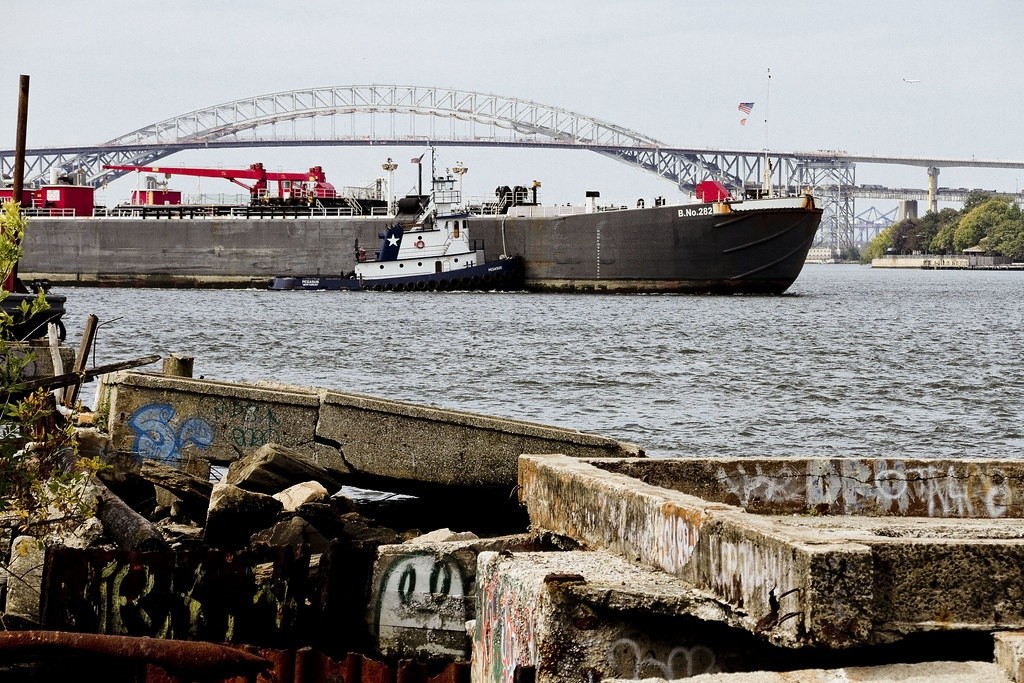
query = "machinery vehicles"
[102,163,335,198]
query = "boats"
[0,76,66,342]
[341,144,525,291]
[390,69,824,294]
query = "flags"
[738,102,754,115]
[739,118,746,125]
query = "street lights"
[382,158,398,216]
[452,160,468,207]
